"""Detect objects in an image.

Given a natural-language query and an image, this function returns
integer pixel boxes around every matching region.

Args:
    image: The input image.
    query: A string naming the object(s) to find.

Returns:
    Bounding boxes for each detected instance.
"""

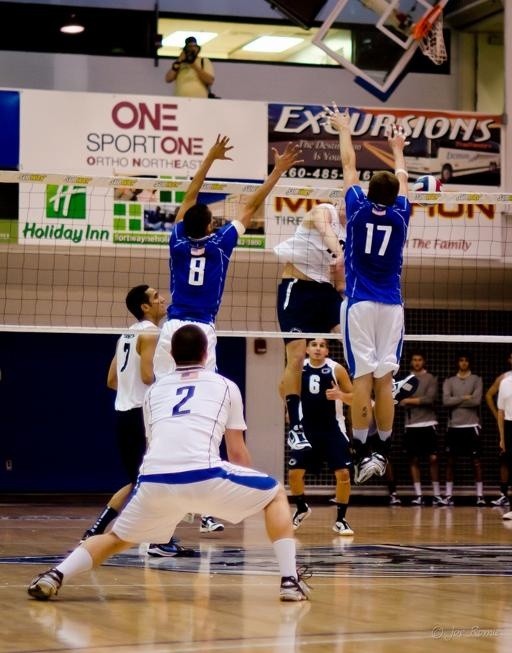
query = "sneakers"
[411,496,425,505]
[279,565,313,601]
[491,496,510,506]
[329,497,338,505]
[333,518,354,535]
[393,375,419,406]
[390,493,402,504]
[200,516,224,533]
[292,504,311,530]
[148,537,194,558]
[287,424,313,451]
[81,527,99,543]
[372,452,389,477]
[353,455,375,485]
[503,511,512,519]
[476,496,487,505]
[433,495,455,506]
[28,567,62,600]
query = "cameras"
[184,46,197,64]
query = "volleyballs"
[413,175,444,207]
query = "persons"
[79,285,195,557]
[329,378,401,506]
[498,371,512,521]
[274,204,419,452]
[200,362,224,533]
[154,134,304,381]
[27,325,316,602]
[399,352,447,507]
[164,37,216,99]
[442,351,487,507]
[487,354,512,507]
[319,101,410,484]
[279,339,355,537]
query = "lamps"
[59,12,85,34]
